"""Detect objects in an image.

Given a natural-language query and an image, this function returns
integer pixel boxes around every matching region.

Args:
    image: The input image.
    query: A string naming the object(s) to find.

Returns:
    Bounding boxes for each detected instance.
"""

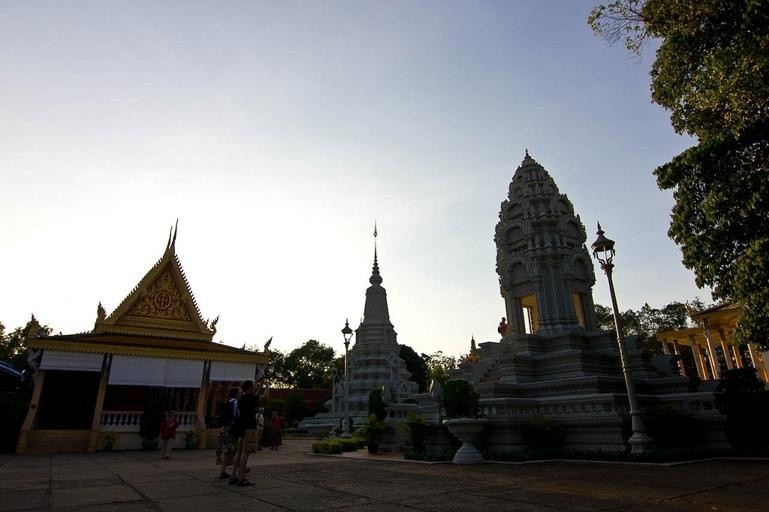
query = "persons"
[500,316,508,337]
[228,380,271,486]
[159,411,181,459]
[216,387,251,478]
[255,407,265,451]
[270,407,287,451]
[230,380,251,474]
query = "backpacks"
[219,399,239,427]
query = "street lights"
[587,217,656,456]
[339,317,354,441]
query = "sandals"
[236,478,256,487]
[219,471,231,479]
[228,476,239,485]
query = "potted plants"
[357,413,385,453]
[94,427,120,451]
[136,400,167,451]
[185,427,201,449]
[396,409,427,459]
[441,375,488,463]
